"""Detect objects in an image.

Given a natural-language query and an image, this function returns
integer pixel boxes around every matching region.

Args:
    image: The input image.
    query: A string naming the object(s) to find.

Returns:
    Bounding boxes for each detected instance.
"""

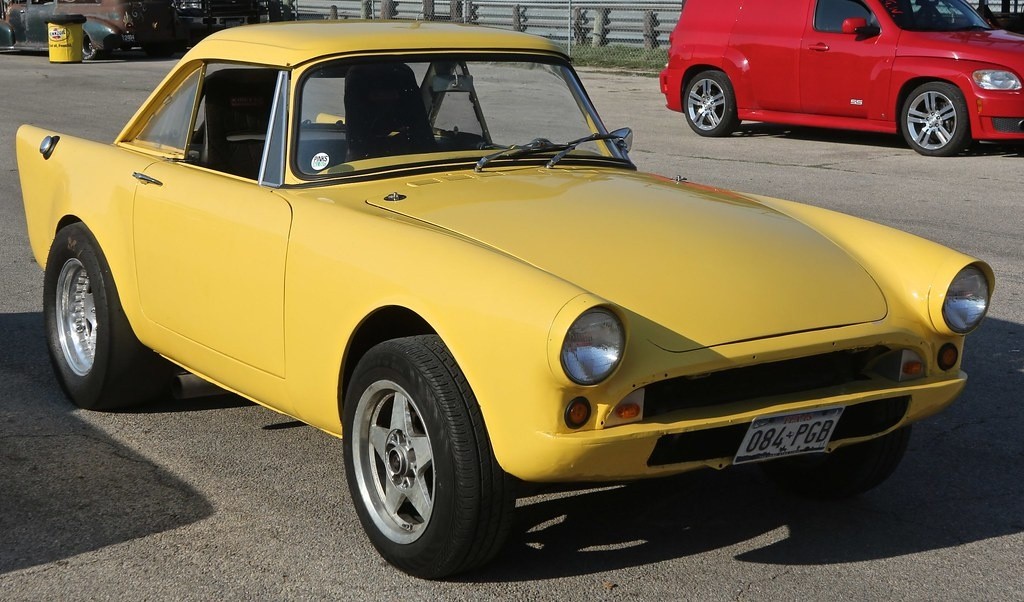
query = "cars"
[13,18,996,584]
[143,0,270,59]
[0,0,157,61]
[659,0,1024,157]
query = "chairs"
[200,62,439,185]
